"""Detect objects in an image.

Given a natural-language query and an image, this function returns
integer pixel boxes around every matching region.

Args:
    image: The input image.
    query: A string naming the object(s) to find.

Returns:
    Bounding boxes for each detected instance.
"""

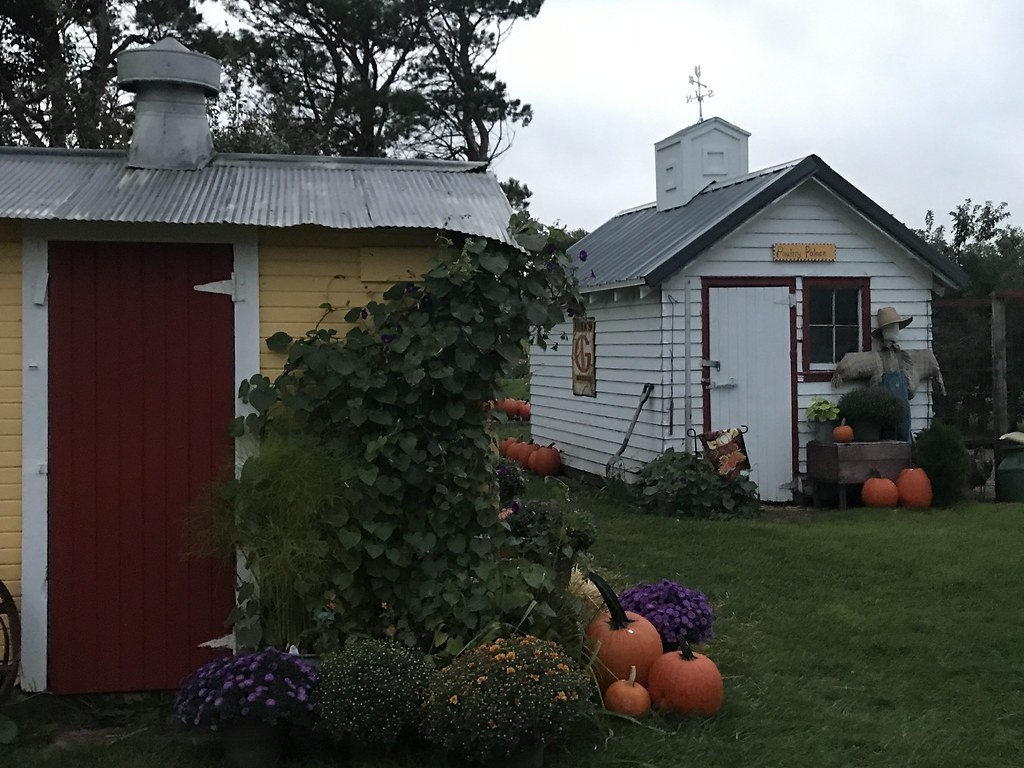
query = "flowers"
[620,578,717,644]
[174,646,319,732]
[310,637,438,743]
[418,633,599,762]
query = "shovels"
[606,382,653,475]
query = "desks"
[806,441,913,509]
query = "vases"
[220,721,283,768]
[471,746,544,768]
[350,741,394,759]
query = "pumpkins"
[499,435,561,477]
[862,469,898,507]
[833,418,853,442]
[605,666,651,717]
[585,571,663,690]
[895,464,933,508]
[495,396,530,416]
[648,634,722,714]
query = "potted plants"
[837,387,908,442]
[640,447,760,517]
[806,397,840,441]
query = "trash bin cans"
[996,445,1024,503]
